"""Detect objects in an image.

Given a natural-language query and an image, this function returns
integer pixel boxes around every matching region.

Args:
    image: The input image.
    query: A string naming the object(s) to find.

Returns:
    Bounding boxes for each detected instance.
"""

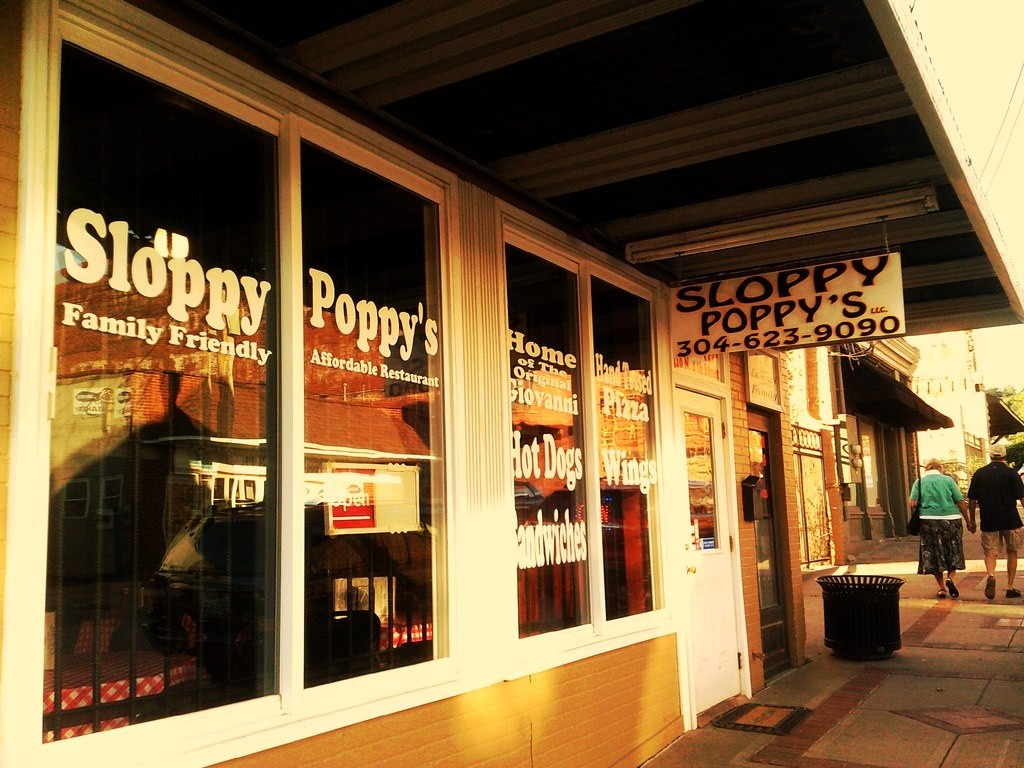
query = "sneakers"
[1005,589,1021,598]
[937,588,946,598]
[945,578,959,598]
[985,576,996,599]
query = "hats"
[989,444,1006,458]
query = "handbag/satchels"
[906,477,922,535]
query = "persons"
[967,443,1024,601]
[905,458,976,598]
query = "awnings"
[839,348,955,435]
[985,392,1024,445]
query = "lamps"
[623,184,942,267]
[851,442,864,470]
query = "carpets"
[710,700,816,738]
[887,703,1024,738]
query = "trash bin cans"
[814,575,905,660]
